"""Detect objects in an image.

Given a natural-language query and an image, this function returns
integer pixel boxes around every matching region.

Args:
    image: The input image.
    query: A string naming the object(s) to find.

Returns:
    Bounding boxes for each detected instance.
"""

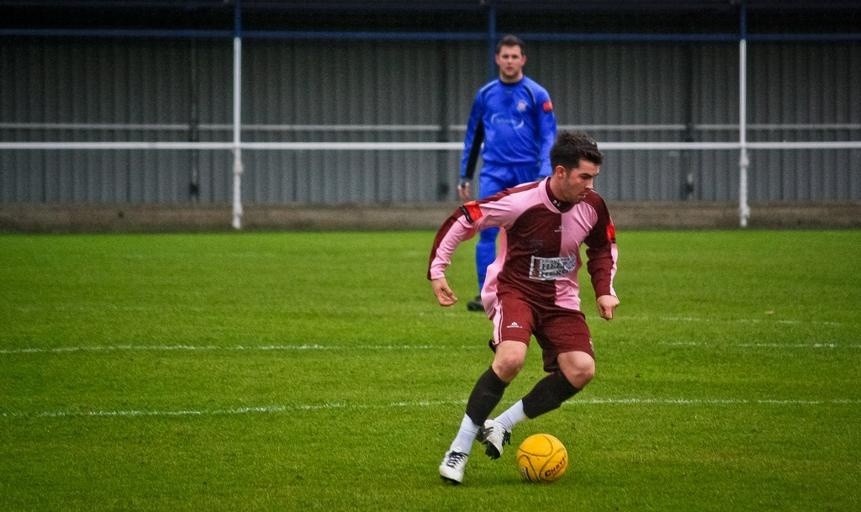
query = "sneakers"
[467,296,484,311]
[476,418,514,460]
[439,450,471,483]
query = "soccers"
[517,433,567,483]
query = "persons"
[458,35,556,312]
[429,130,619,486]
